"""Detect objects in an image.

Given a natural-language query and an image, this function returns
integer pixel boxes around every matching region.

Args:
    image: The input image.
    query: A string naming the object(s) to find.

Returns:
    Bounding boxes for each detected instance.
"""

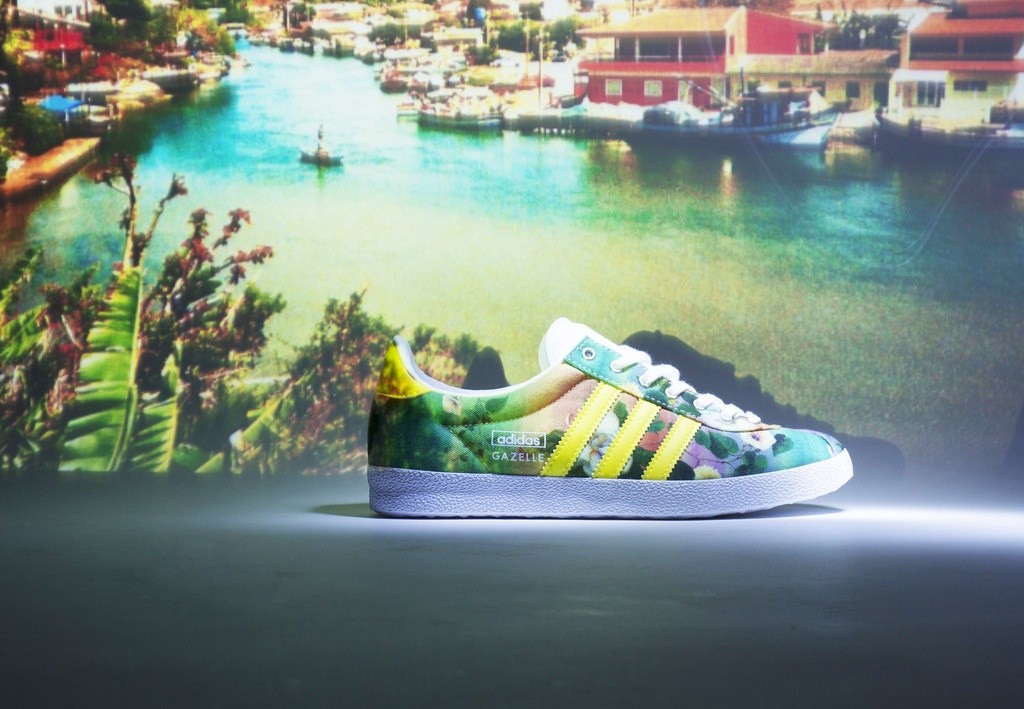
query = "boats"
[253,24,510,126]
[0,26,231,208]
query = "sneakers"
[366,317,854,520]
